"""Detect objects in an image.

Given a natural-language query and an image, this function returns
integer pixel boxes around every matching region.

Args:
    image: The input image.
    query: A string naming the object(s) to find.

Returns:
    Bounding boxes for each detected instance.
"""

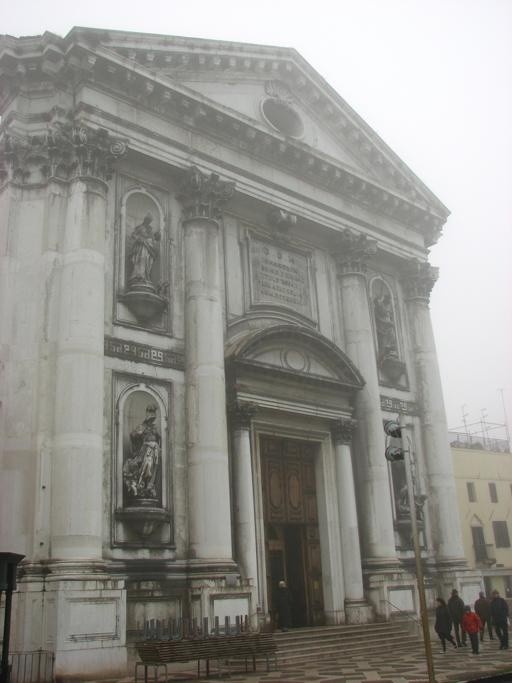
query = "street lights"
[382,402,437,682]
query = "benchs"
[136,638,277,683]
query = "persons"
[129,213,161,283]
[489,589,510,650]
[435,599,457,654]
[474,592,496,641]
[448,590,468,647]
[278,581,293,632]
[462,605,483,653]
[374,293,396,356]
[122,415,163,499]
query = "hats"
[493,591,499,594]
[464,606,470,610]
[452,589,457,593]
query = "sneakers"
[440,642,467,654]
[472,649,479,654]
[500,643,508,648]
[479,637,496,641]
